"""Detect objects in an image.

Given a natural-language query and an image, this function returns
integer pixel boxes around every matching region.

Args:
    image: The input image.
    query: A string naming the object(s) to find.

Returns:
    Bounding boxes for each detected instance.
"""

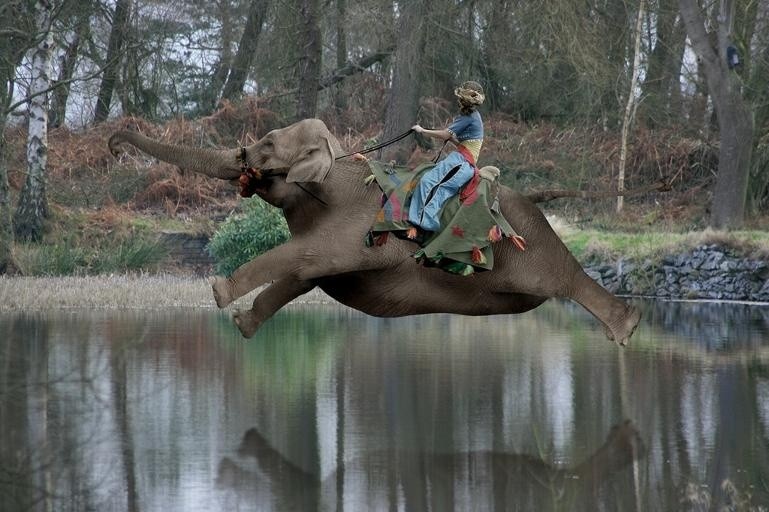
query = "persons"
[401,81,486,235]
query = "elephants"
[108,119,665,350]
[214,419,647,512]
